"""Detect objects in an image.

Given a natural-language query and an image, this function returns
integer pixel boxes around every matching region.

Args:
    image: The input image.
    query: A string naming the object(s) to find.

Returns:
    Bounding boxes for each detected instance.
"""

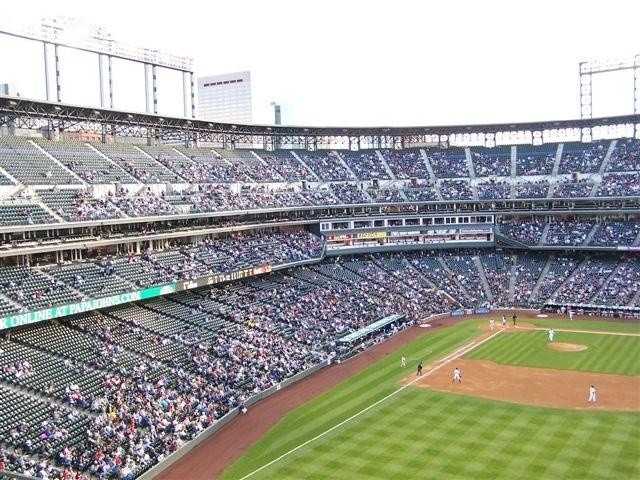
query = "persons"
[0,140,639,480]
